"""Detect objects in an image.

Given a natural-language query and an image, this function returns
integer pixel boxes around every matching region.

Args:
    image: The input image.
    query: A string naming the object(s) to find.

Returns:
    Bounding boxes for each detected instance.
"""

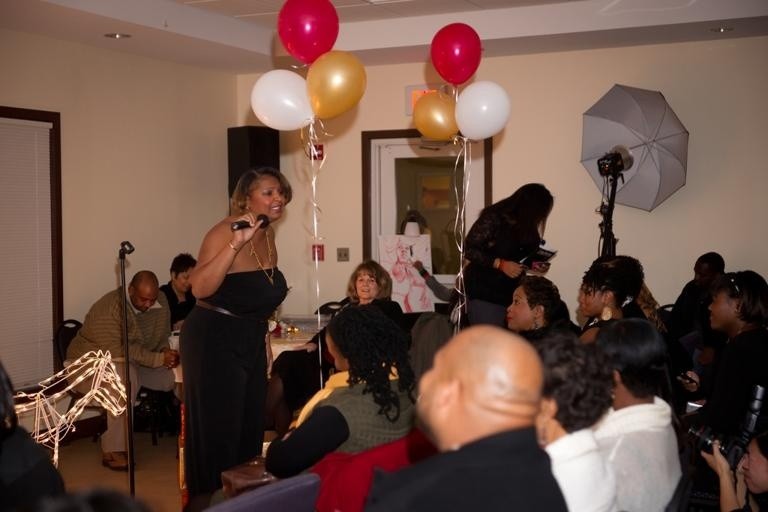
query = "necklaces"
[249,232,279,286]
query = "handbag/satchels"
[221,455,279,500]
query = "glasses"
[720,272,741,297]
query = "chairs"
[54,319,160,446]
[314,300,343,314]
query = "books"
[517,247,557,269]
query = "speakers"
[227,125,281,201]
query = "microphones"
[230,214,270,230]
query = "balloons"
[428,21,483,86]
[302,46,368,123]
[410,90,460,143]
[274,0,340,67]
[452,81,513,144]
[249,67,316,134]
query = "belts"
[195,299,242,318]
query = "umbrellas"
[578,82,690,214]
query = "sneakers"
[102,451,128,470]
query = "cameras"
[683,382,766,471]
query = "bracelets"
[226,237,239,256]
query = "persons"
[461,182,560,331]
[178,168,290,512]
[64,268,179,472]
[258,252,768,512]
[157,253,201,331]
[393,208,431,238]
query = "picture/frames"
[404,83,446,116]
[414,171,455,213]
